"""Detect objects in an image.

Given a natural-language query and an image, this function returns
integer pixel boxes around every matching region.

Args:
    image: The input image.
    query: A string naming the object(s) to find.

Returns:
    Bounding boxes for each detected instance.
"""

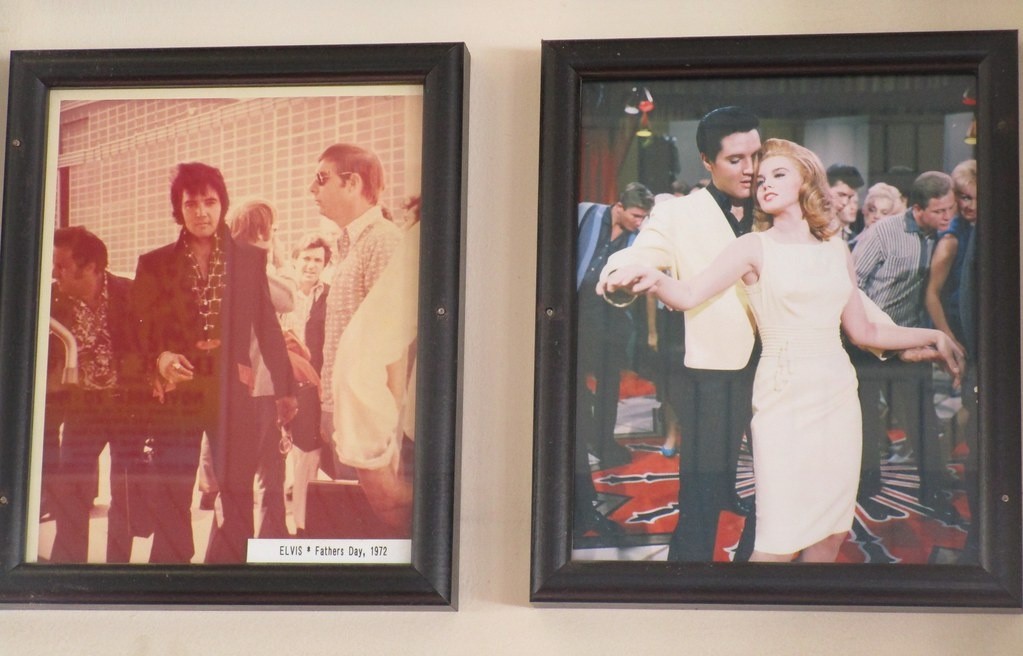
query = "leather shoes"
[919,487,959,519]
[720,495,752,516]
[591,512,625,539]
[857,469,881,498]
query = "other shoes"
[662,445,677,457]
[200,491,218,510]
[587,440,629,466]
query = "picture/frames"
[1,42,467,604]
[540,28,1021,608]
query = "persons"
[33,143,422,565]
[573,105,977,566]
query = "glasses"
[316,171,352,186]
